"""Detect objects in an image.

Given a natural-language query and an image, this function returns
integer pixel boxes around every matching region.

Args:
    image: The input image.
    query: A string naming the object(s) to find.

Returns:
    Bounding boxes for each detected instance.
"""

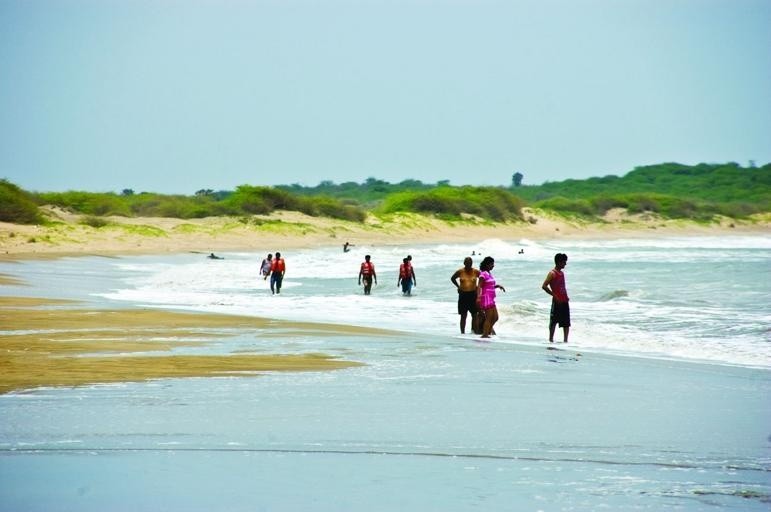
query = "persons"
[263,252,285,295]
[542,253,571,342]
[478,257,505,339]
[259,254,272,276]
[397,258,417,296]
[450,257,481,334]
[406,255,412,266]
[359,255,377,295]
